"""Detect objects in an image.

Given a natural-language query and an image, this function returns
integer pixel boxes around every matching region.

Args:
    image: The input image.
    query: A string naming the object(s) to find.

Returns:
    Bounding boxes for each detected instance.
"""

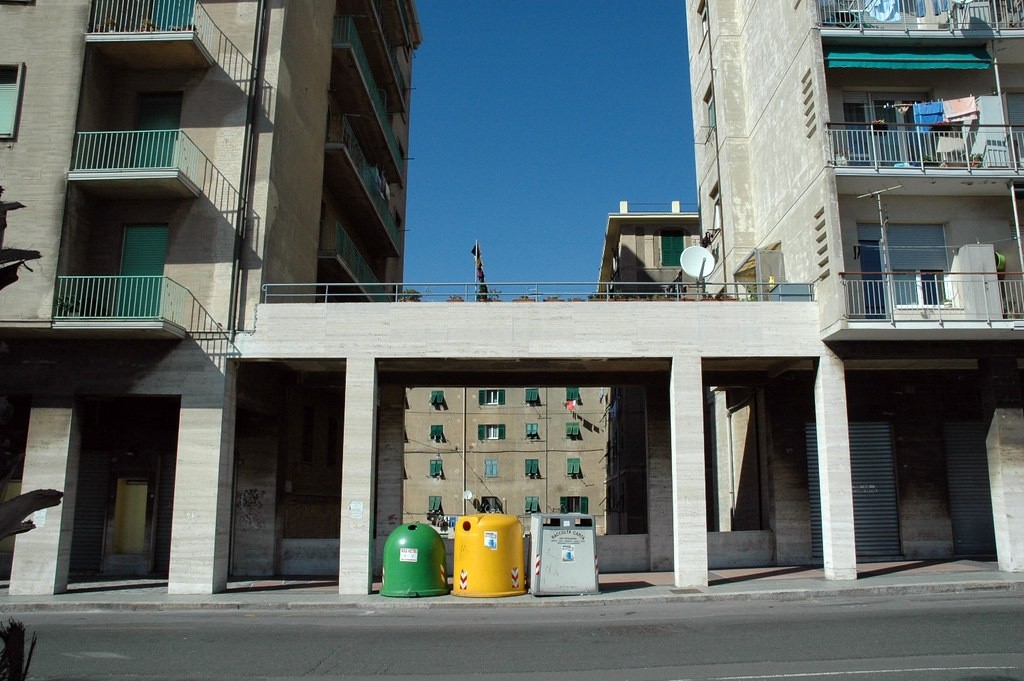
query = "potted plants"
[929,122,952,137]
[869,119,889,135]
[447,288,738,302]
[401,289,421,302]
[835,147,847,167]
[968,153,984,167]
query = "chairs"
[936,136,964,166]
[916,12,948,30]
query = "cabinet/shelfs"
[957,243,1003,321]
[949,0,992,29]
[965,95,1008,169]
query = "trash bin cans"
[450,513,527,598]
[379,520,451,598]
[526,513,602,596]
